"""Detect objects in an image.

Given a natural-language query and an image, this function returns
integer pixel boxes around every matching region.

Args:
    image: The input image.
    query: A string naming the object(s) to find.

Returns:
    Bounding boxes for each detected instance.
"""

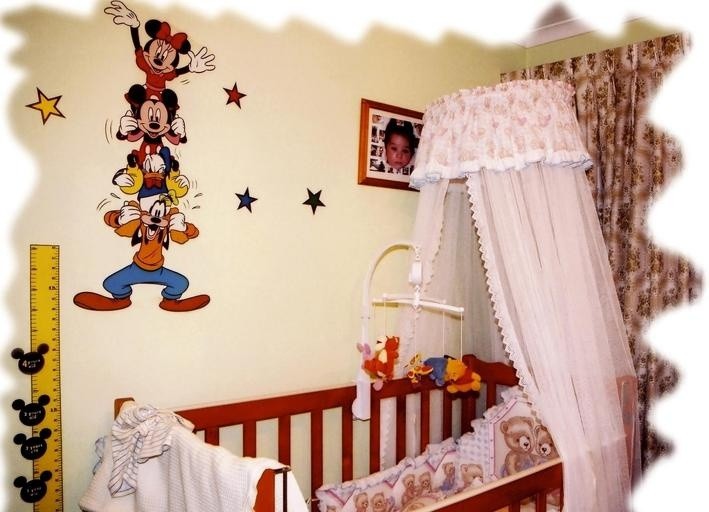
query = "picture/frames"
[357,98,426,192]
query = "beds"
[114,352,637,512]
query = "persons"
[384,127,413,168]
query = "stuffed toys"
[361,329,484,396]
